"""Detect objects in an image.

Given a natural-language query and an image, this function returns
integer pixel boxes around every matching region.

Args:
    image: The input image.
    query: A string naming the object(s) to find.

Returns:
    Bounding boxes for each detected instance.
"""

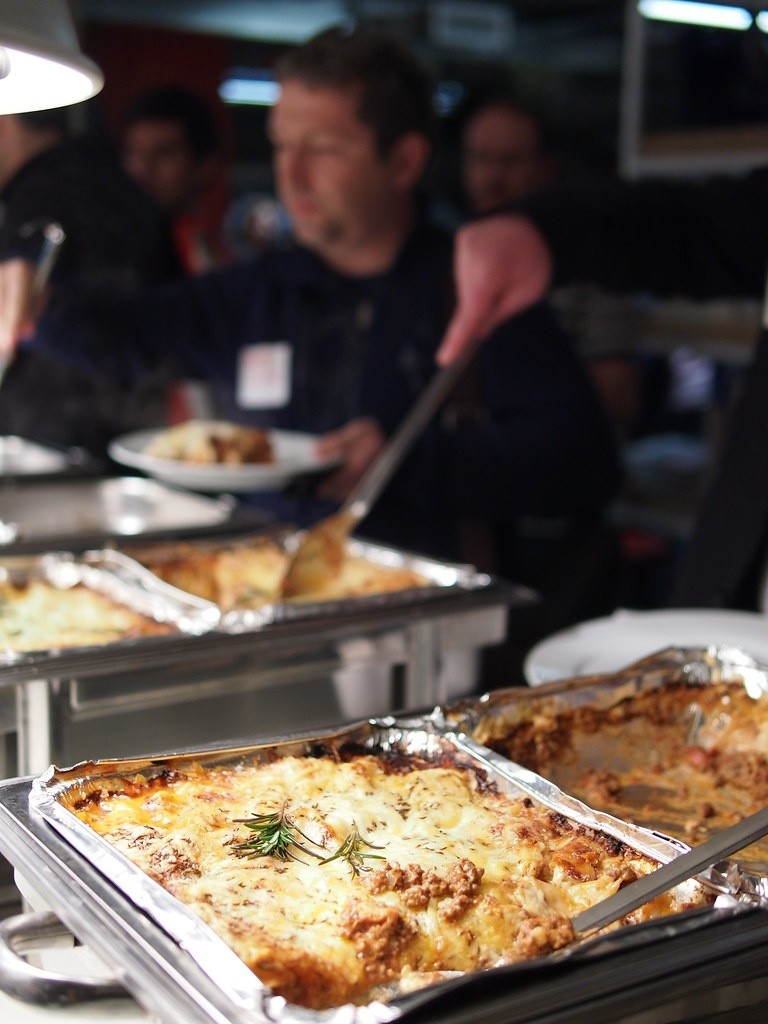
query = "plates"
[112,427,346,489]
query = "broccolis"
[230,799,388,882]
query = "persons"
[1,24,768,689]
